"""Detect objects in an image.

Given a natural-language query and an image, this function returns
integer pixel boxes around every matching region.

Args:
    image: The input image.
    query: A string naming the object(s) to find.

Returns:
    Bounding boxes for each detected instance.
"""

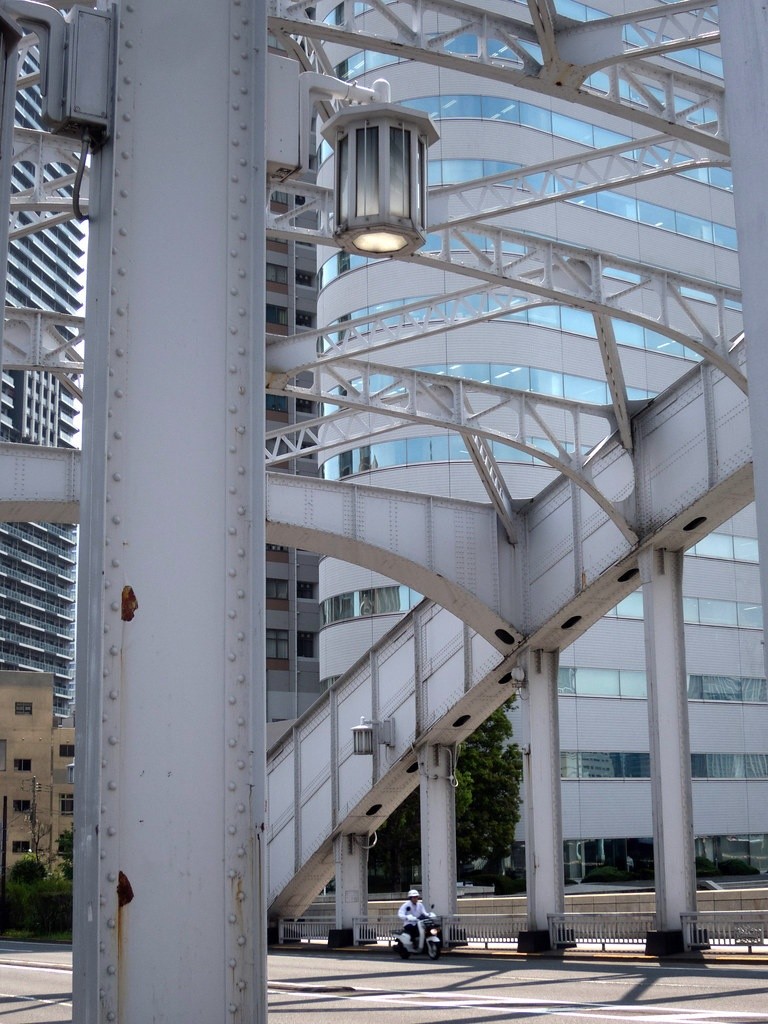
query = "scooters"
[395,903,444,961]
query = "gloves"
[430,913,435,917]
[407,915,416,922]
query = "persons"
[398,889,435,949]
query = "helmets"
[407,890,421,897]
